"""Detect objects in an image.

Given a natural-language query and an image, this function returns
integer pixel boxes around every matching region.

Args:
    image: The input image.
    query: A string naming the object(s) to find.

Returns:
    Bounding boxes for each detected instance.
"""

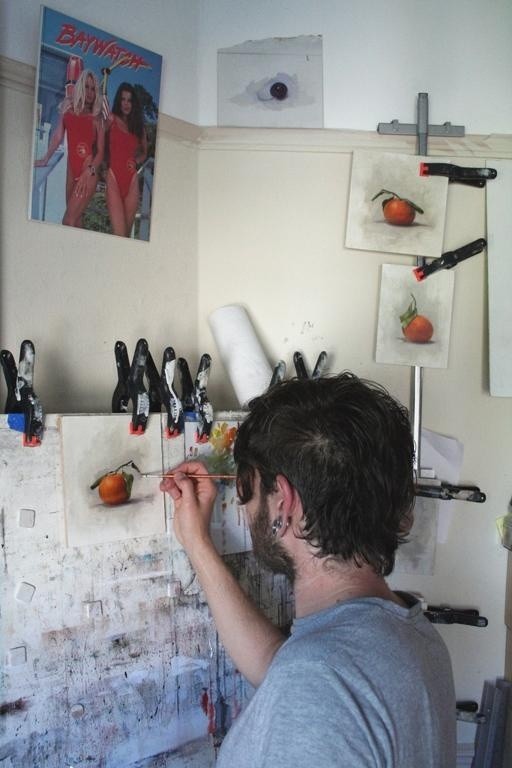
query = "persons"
[34,68,106,228]
[102,79,150,237]
[159,367,458,768]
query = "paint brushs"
[140,474,238,479]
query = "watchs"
[86,164,97,176]
